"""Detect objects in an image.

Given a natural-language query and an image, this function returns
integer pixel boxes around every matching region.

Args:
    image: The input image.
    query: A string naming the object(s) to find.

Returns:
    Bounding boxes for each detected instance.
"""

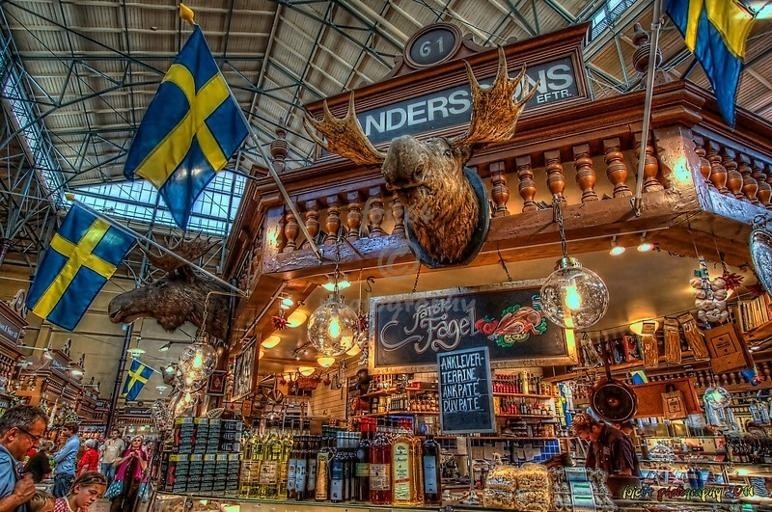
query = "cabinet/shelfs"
[345,353,772,512]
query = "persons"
[573,413,642,477]
[0,404,152,512]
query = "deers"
[107,228,234,343]
[302,43,539,271]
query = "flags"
[121,360,154,401]
[25,203,137,332]
[666,1,757,131]
[124,25,250,231]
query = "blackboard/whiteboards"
[437,346,496,436]
[366,278,577,377]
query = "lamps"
[636,236,654,252]
[610,240,626,256]
[48,366,82,377]
[16,344,52,360]
[628,320,660,337]
[537,198,610,329]
[164,239,365,414]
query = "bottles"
[725,434,751,464]
[492,370,552,415]
[386,391,440,412]
[239,423,442,506]
